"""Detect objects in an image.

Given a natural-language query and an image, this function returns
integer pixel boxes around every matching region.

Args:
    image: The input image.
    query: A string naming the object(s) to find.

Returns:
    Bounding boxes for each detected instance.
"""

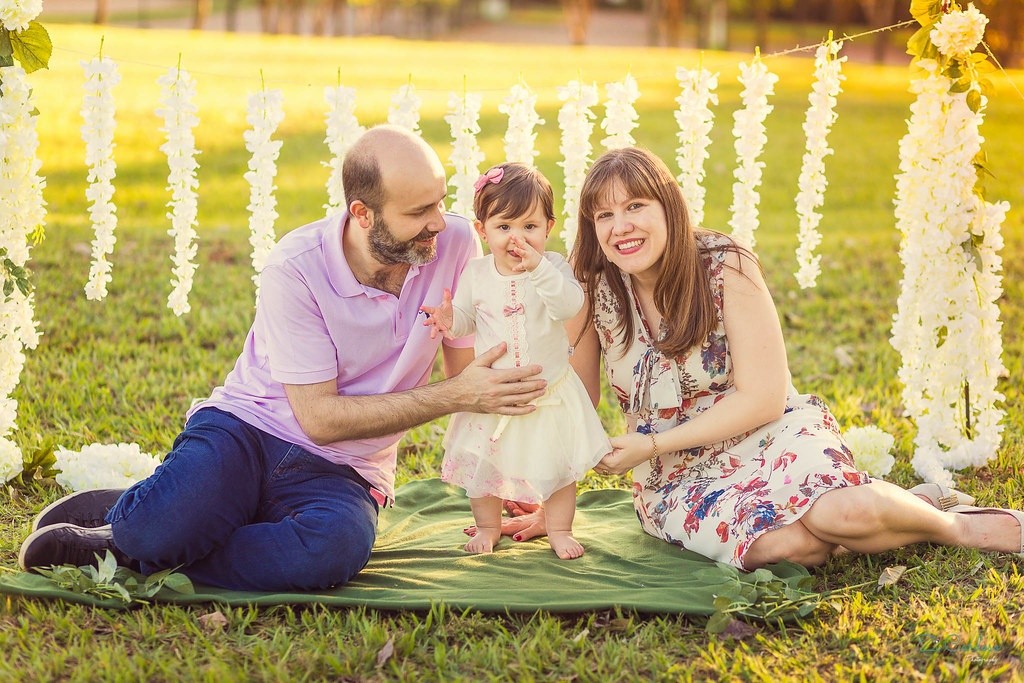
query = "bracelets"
[650,434,657,468]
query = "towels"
[0,477,812,623]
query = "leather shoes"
[907,484,976,512]
[946,505,1024,557]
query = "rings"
[602,469,608,475]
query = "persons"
[462,146,1024,574]
[419,163,616,559]
[17,127,543,593]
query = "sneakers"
[31,489,128,533]
[18,522,141,575]
